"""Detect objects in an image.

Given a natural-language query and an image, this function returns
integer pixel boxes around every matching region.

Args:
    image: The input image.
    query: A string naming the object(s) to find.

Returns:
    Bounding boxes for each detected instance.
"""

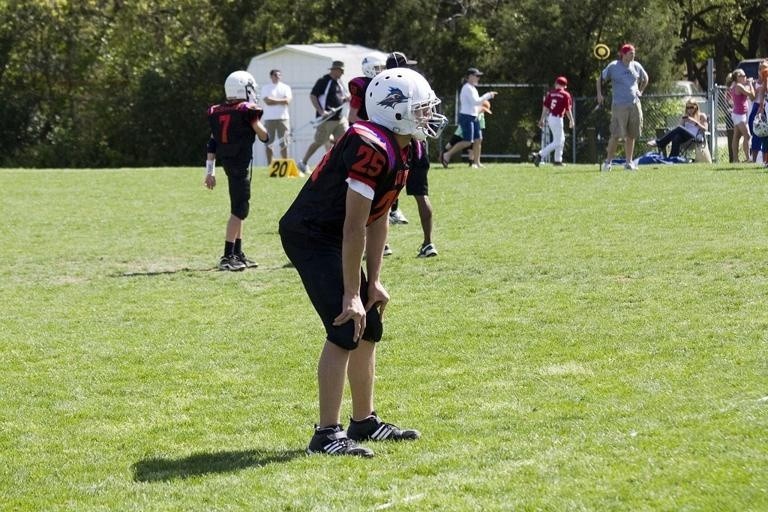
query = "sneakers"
[217,253,246,271]
[347,409,421,442]
[389,208,408,224]
[383,243,392,255]
[307,424,375,459]
[624,162,636,170]
[236,252,258,268]
[647,140,657,147]
[603,162,611,172]
[297,159,313,175]
[533,152,541,168]
[416,242,438,257]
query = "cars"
[650,81,709,130]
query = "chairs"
[678,112,711,161]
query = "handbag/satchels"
[752,112,768,138]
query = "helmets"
[362,54,385,78]
[365,66,449,143]
[224,69,261,105]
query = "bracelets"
[205,159,215,178]
[694,122,699,126]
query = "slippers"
[439,150,449,168]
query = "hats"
[555,76,568,85]
[619,44,635,54]
[467,67,484,75]
[386,51,418,68]
[327,61,344,70]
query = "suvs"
[724,57,764,128]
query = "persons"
[594,43,650,174]
[259,68,291,167]
[359,51,438,261]
[646,98,709,165]
[724,70,756,163]
[197,69,275,271]
[343,55,410,226]
[296,61,352,179]
[270,65,448,455]
[532,75,576,168]
[750,59,768,164]
[444,78,487,167]
[439,67,500,170]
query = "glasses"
[686,106,694,109]
